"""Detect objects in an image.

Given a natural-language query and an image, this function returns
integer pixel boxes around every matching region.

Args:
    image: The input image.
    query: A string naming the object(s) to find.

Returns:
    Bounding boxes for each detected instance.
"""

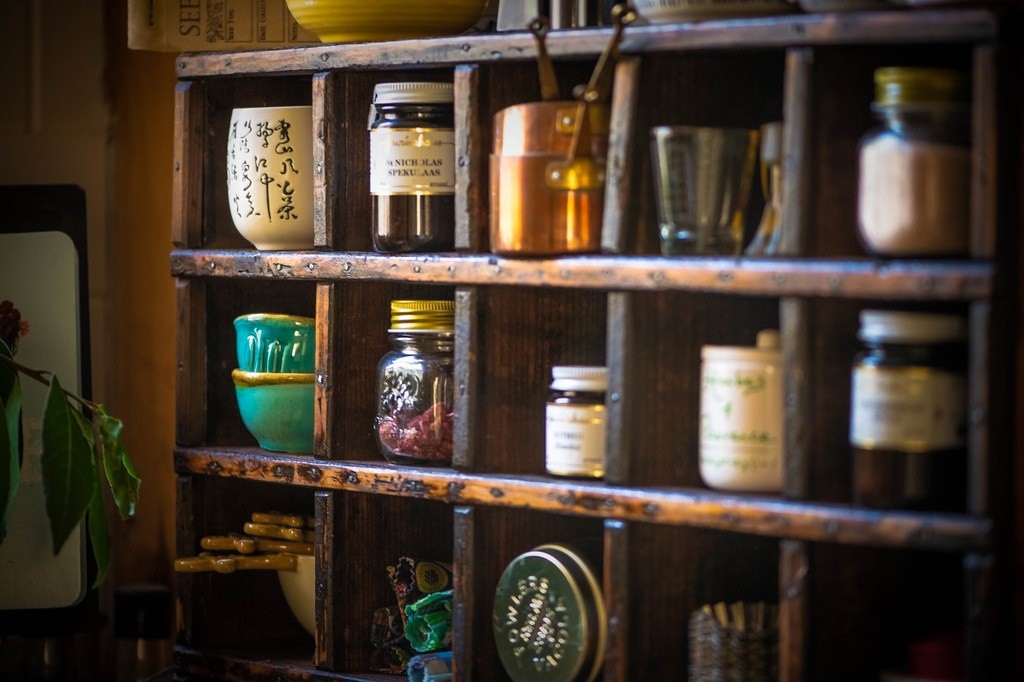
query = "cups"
[699,346,783,491]
[652,127,758,257]
[227,106,313,251]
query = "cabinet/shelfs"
[173,9,1001,681]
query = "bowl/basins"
[232,370,315,455]
[232,314,315,373]
[634,0,790,24]
[286,0,486,44]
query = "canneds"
[848,312,965,511]
[370,81,456,253]
[859,66,971,256]
[544,365,608,478]
[376,299,456,467]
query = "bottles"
[375,301,456,465]
[860,67,970,258]
